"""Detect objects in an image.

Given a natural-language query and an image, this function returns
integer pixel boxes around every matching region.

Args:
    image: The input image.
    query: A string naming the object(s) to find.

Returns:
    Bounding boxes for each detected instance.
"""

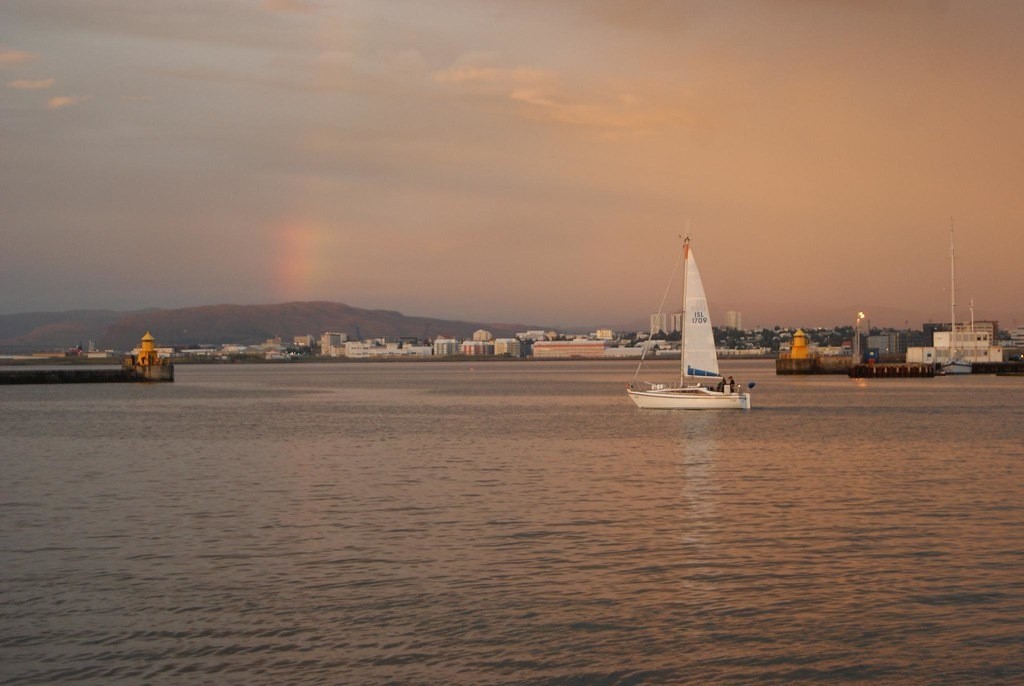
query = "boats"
[122,328,175,383]
[774,327,818,375]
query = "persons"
[728,376,736,393]
[716,379,727,392]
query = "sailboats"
[939,214,973,375]
[626,229,754,412]
